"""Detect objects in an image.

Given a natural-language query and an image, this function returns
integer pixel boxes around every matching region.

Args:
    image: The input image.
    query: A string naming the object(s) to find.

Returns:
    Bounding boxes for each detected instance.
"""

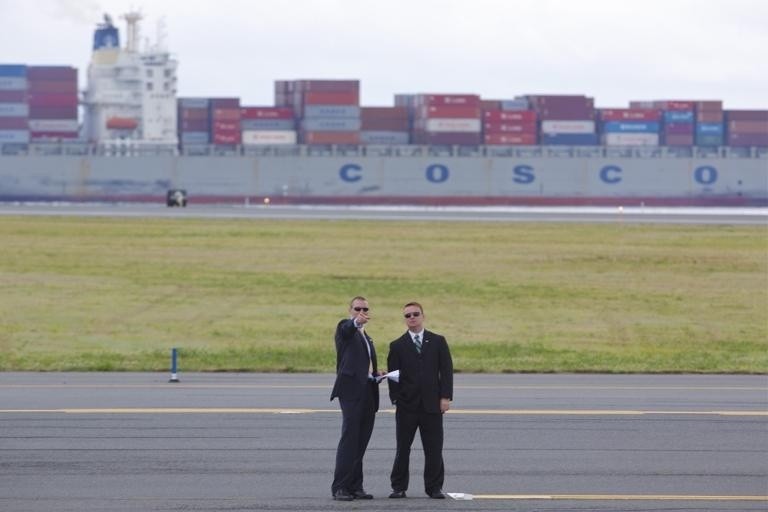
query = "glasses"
[404,312,420,317]
[354,307,369,312]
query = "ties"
[414,335,422,352]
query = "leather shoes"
[431,492,444,499]
[388,491,406,498]
[353,491,373,499]
[334,490,353,501]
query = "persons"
[330,297,388,500]
[386,302,453,499]
